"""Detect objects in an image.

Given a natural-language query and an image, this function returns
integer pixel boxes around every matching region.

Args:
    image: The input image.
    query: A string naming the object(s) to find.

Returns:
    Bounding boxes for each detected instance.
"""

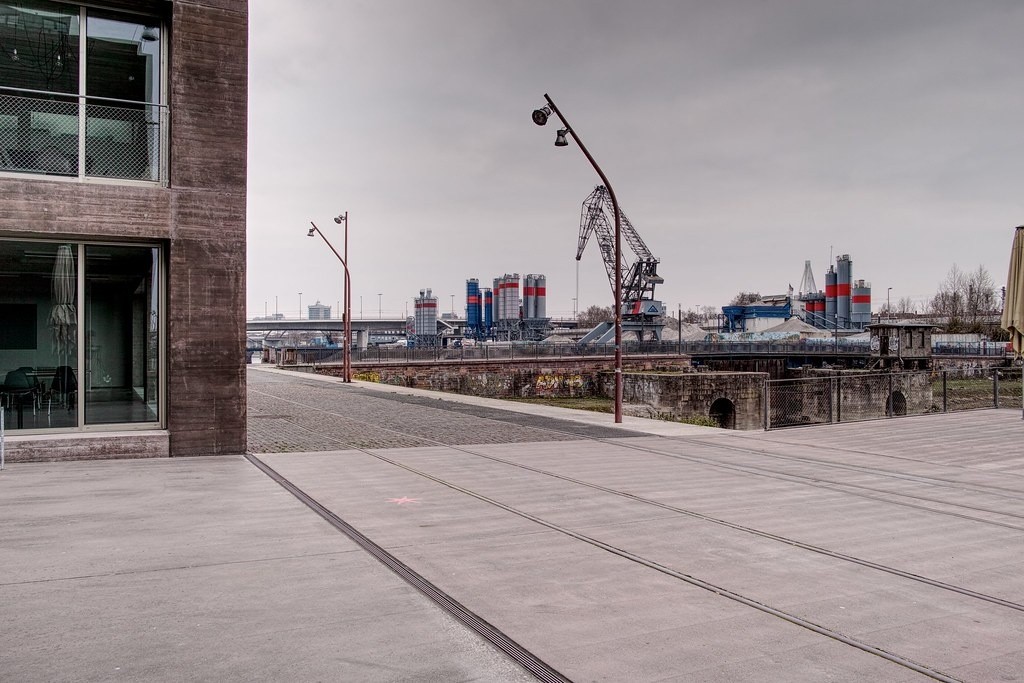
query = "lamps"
[55,29,63,68]
[11,22,19,62]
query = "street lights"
[334,211,348,383]
[531,93,622,423]
[834,312,838,353]
[307,222,352,383]
[887,287,892,323]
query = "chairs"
[0,365,80,427]
